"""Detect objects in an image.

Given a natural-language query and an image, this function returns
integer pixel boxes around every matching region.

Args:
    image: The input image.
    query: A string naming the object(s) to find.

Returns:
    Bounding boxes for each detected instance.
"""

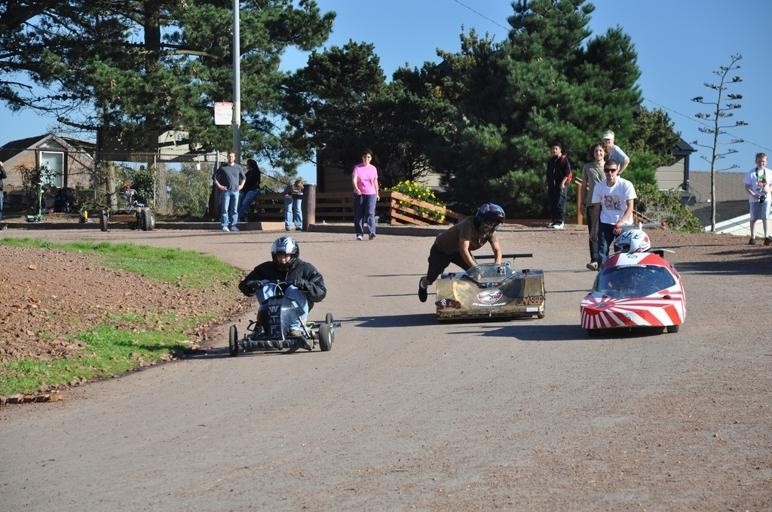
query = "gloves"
[247,279,263,291]
[292,276,311,289]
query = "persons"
[0,161,7,230]
[211,151,247,233]
[744,152,772,246]
[351,148,380,241]
[238,158,261,222]
[282,180,305,231]
[238,236,327,336]
[578,129,651,298]
[545,142,573,230]
[417,201,506,303]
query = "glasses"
[604,168,617,173]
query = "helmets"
[270,235,301,268]
[612,227,652,255]
[475,203,506,233]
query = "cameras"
[760,196,764,203]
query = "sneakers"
[417,276,429,303]
[231,226,239,231]
[762,238,772,246]
[356,236,363,241]
[548,221,564,230]
[254,332,270,340]
[585,261,598,271]
[368,233,376,241]
[748,239,756,245]
[287,330,302,336]
[222,226,230,232]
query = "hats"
[601,130,615,140]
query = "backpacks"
[559,154,573,185]
